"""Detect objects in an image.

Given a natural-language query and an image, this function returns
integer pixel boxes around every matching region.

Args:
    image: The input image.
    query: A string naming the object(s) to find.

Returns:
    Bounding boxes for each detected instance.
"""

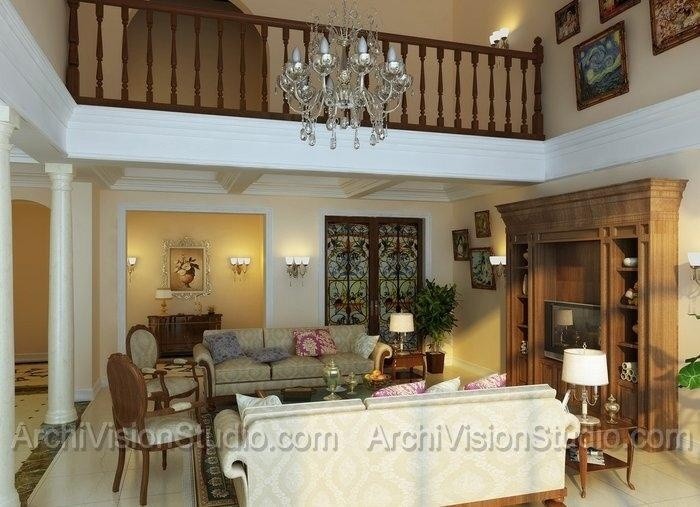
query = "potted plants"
[413,276,464,374]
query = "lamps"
[485,25,511,49]
[230,256,237,267]
[294,256,301,266]
[273,2,415,151]
[238,257,244,266]
[387,311,416,356]
[285,256,294,267]
[154,286,174,317]
[561,346,611,428]
[301,256,309,267]
[244,257,251,266]
[500,255,507,277]
[489,255,503,279]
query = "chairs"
[105,352,202,507]
[125,322,201,421]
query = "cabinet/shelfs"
[147,313,224,358]
[494,177,690,454]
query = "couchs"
[212,381,582,507]
[191,322,394,390]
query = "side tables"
[387,346,428,383]
[576,414,640,499]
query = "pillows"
[203,329,248,367]
[463,372,508,391]
[243,343,294,364]
[370,377,427,399]
[424,376,461,393]
[291,326,340,358]
[234,392,283,418]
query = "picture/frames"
[554,2,581,44]
[648,0,698,58]
[597,0,642,24]
[570,17,631,110]
[469,246,496,291]
[156,233,213,301]
[474,209,492,239]
[451,228,470,262]
